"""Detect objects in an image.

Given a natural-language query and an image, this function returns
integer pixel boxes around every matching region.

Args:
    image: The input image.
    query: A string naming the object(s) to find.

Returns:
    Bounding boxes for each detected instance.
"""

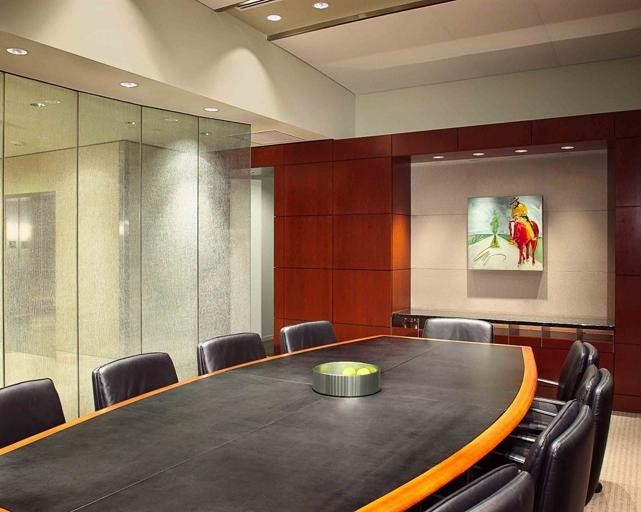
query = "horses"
[508,219,540,268]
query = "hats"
[509,197,519,206]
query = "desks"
[1,336,536,510]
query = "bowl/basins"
[311,360,381,398]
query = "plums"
[319,364,333,374]
[341,366,376,376]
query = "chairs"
[2,379,67,450]
[421,319,494,342]
[198,333,268,377]
[510,339,598,434]
[503,368,612,508]
[402,464,534,512]
[280,320,336,356]
[91,350,179,414]
[453,400,595,509]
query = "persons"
[510,197,535,245]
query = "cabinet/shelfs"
[392,327,615,394]
